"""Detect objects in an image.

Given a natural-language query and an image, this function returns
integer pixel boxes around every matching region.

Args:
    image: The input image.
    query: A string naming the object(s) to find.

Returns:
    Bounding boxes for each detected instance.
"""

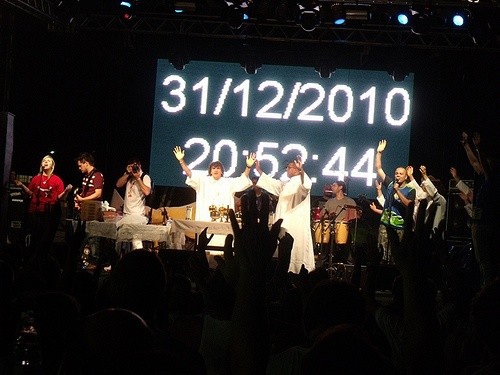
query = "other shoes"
[104,265,112,271]
[86,264,96,269]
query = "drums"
[312,220,331,244]
[334,220,350,244]
[311,207,322,220]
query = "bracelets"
[180,160,184,164]
[376,151,382,154]
[299,168,304,173]
[246,165,251,168]
[136,177,141,181]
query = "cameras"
[132,162,139,173]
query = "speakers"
[446,178,475,239]
[159,248,209,276]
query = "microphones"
[394,180,398,189]
[348,204,362,209]
[42,161,45,168]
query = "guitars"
[74,188,81,215]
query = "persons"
[9,169,25,203]
[406,165,447,256]
[459,131,500,298]
[14,155,73,214]
[241,177,270,229]
[318,181,357,265]
[375,139,417,267]
[310,183,336,213]
[116,159,152,250]
[172,145,257,255]
[310,164,487,283]
[0,188,500,375]
[450,166,473,219]
[255,155,316,274]
[74,153,104,268]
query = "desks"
[72,219,287,257]
